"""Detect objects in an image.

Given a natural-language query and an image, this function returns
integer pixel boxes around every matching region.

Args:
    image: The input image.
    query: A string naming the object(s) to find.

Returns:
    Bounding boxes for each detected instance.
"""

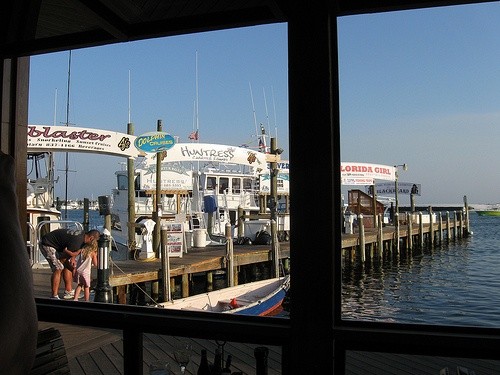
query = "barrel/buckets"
[194,229,207,247]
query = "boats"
[152,275,290,316]
[110,52,395,251]
[28,88,139,268]
[476,210,500,217]
[55,194,99,210]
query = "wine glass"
[172,342,193,375]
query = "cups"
[149,360,171,375]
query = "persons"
[69,241,98,302]
[39,227,100,299]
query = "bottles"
[197,349,231,375]
[254,346,269,375]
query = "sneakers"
[55,294,61,300]
[64,290,84,299]
